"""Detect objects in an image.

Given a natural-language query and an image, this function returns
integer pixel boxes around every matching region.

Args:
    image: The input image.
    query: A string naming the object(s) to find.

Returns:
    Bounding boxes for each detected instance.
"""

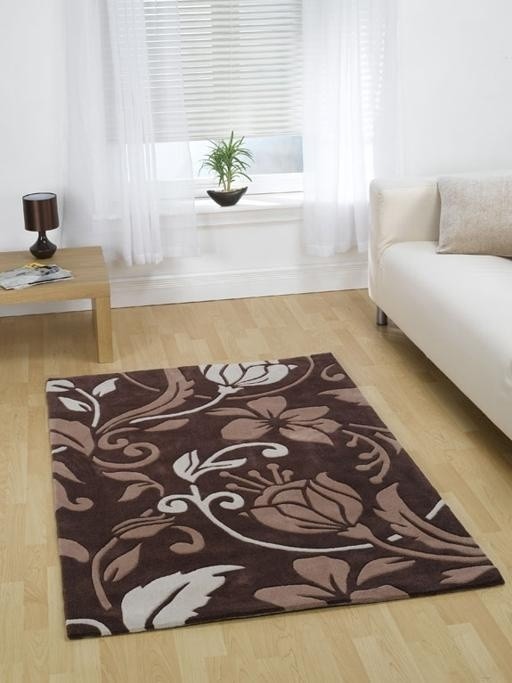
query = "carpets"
[44,353,506,640]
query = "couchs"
[367,169,512,442]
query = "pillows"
[436,173,512,258]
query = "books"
[1,262,73,290]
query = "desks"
[0,246,114,363]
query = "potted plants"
[197,131,255,207]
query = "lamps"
[23,192,59,259]
